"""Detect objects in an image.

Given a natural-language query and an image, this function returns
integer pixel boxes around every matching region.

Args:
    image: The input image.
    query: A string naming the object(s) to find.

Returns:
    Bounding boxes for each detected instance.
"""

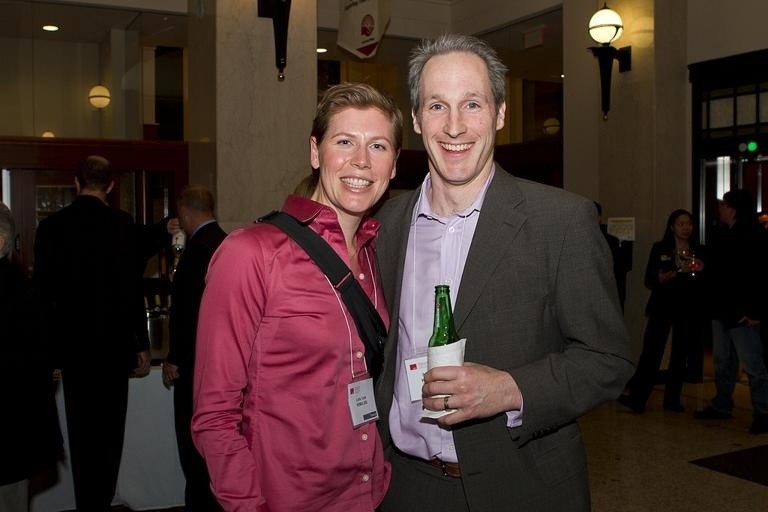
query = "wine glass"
[687,254,697,278]
[676,249,689,273]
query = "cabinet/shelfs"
[0,136,209,366]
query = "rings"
[444,396,451,411]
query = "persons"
[162,185,229,512]
[191,82,393,512]
[33,154,150,511]
[370,34,638,511]
[592,201,626,318]
[616,208,702,414]
[692,189,767,435]
[0,200,69,512]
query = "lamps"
[88,86,112,109]
[542,118,560,136]
[586,2,631,120]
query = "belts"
[417,459,463,477]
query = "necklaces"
[678,242,688,251]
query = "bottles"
[429,283,458,346]
[165,228,186,274]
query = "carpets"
[688,445,768,486]
